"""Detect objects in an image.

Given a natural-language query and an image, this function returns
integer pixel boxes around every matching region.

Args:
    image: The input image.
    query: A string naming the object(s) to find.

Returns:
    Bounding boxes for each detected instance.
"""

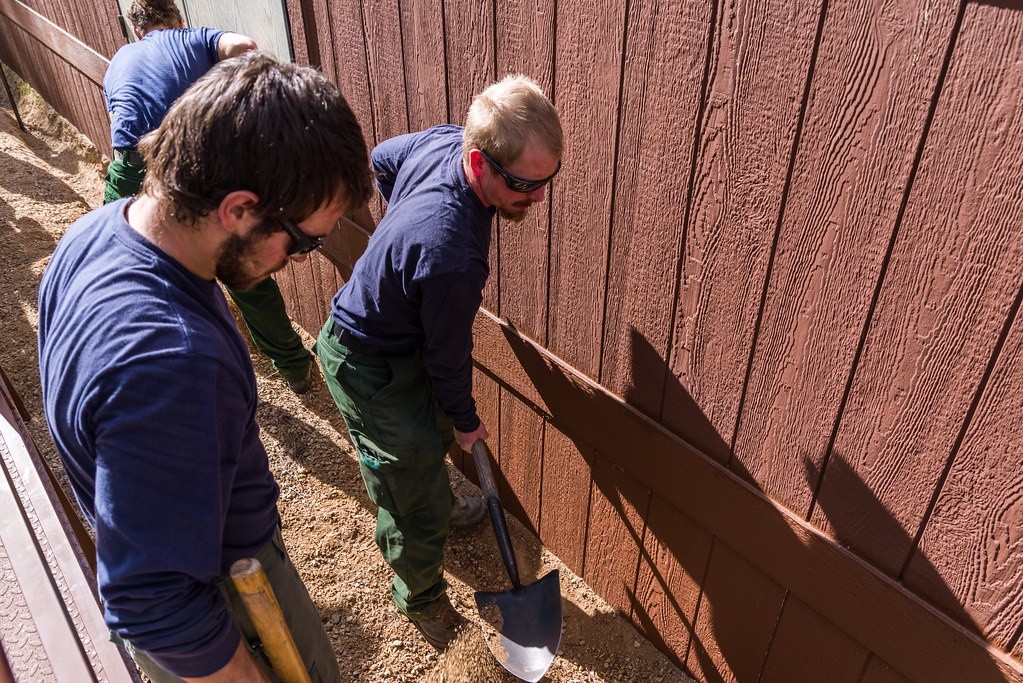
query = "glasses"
[270,208,324,258]
[474,143,563,194]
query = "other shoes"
[287,356,312,393]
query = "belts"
[112,149,146,165]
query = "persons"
[37,52,375,683]
[103,0,313,394]
[316,77,563,650]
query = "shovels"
[471,438,562,683]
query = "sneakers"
[395,593,466,649]
[447,495,487,526]
